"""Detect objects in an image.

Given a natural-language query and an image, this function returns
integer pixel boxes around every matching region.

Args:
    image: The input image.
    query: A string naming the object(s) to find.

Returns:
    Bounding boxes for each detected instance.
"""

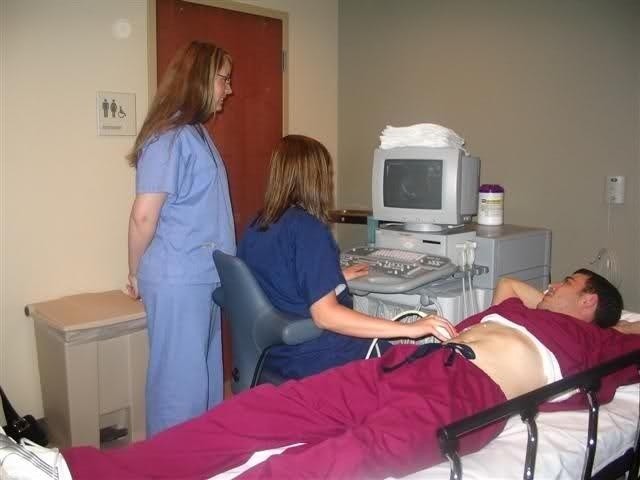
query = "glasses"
[213,71,231,81]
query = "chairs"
[211,250,324,397]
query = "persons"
[122,43,234,439]
[234,135,459,384]
[1,268,640,479]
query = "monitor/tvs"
[372,146,481,232]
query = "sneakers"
[1,427,73,480]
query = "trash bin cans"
[25,290,149,450]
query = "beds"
[205,308,640,479]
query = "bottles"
[478,184,504,226]
